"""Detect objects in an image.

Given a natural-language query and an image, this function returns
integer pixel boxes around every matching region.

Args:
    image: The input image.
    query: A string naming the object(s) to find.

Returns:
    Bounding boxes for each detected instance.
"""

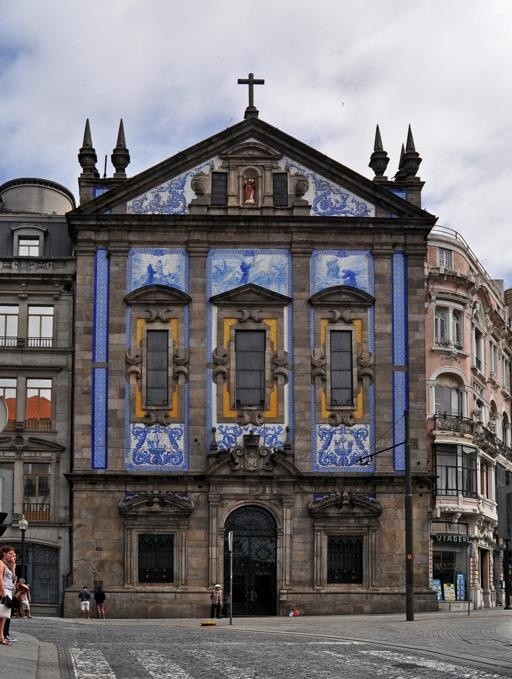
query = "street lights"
[18,513,29,577]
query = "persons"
[0,544,32,646]
[209,584,224,618]
[78,585,92,619]
[94,586,106,619]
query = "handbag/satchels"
[0,603,12,618]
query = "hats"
[215,585,220,587]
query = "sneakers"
[0,635,16,646]
